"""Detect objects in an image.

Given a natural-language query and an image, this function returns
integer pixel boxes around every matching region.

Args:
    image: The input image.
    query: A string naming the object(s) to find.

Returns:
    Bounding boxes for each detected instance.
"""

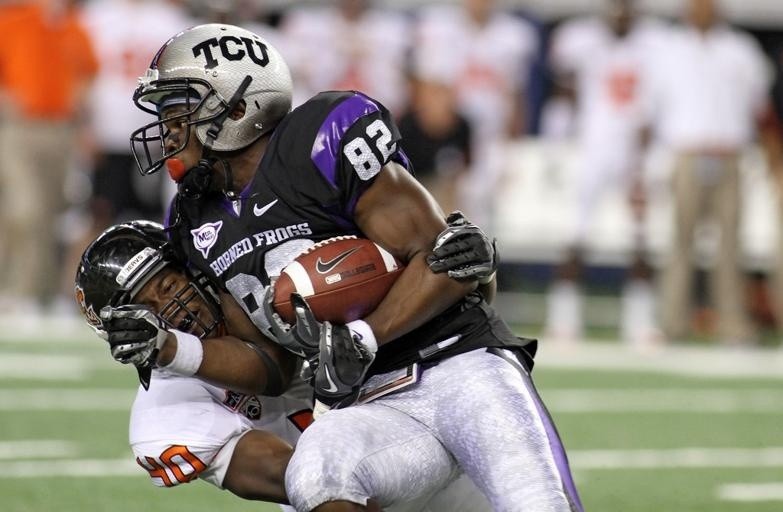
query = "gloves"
[263,286,373,410]
[426,210,501,284]
[99,304,169,391]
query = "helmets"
[75,219,224,342]
[130,23,294,177]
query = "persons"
[75,22,582,512]
[0,0,783,348]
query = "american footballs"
[271,236,405,322]
